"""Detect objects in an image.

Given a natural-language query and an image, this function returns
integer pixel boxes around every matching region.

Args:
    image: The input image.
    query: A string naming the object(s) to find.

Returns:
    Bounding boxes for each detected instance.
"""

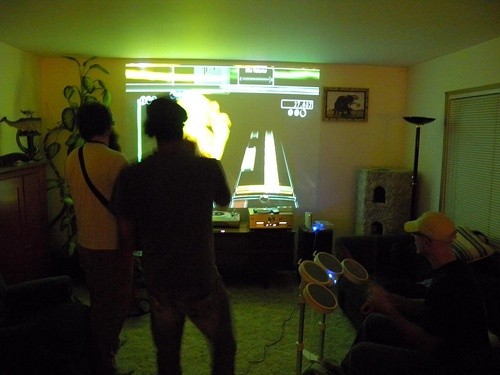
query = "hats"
[147,99,189,122]
[404,212,457,241]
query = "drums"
[313,250,345,277]
[302,283,338,312]
[298,260,330,284]
[342,259,368,284]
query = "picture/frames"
[322,86,370,123]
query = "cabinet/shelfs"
[210,220,296,280]
[0,161,56,291]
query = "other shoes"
[303,356,343,375]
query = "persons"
[64,101,131,375]
[324,212,492,375]
[110,97,236,375]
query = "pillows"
[450,224,497,265]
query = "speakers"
[296,227,333,287]
